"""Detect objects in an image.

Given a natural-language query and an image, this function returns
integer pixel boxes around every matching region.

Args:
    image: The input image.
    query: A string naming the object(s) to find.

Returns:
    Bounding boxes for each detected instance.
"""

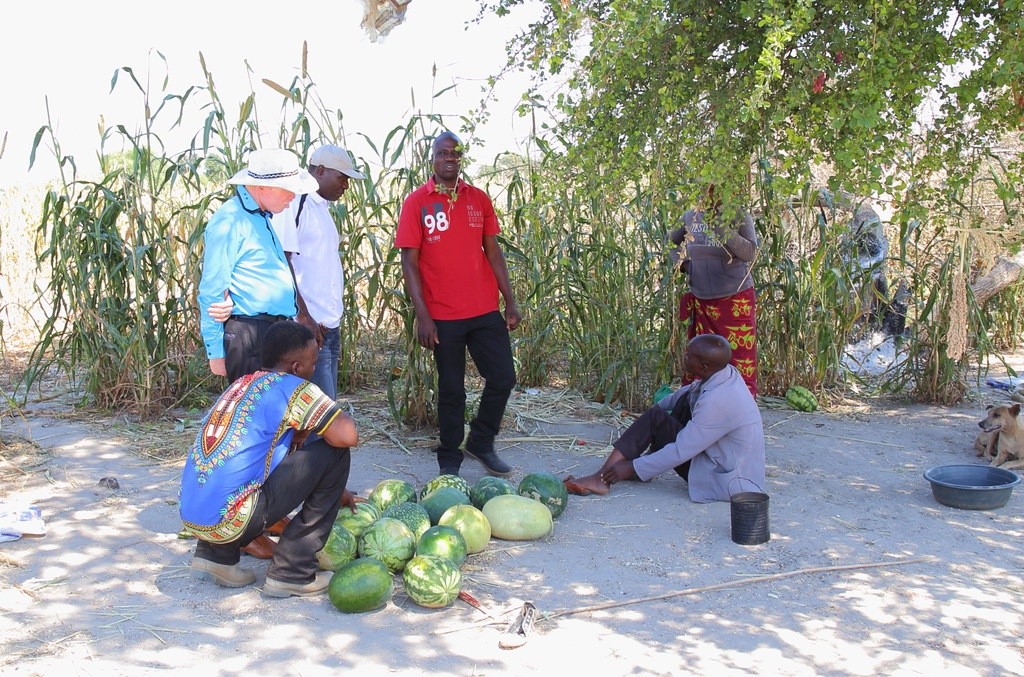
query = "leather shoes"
[261,515,293,537]
[239,533,280,559]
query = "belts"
[237,313,294,324]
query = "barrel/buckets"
[728,477,770,545]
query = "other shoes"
[189,556,257,588]
[501,599,537,648]
[92,477,120,494]
[464,433,512,477]
[439,448,464,477]
[264,571,335,598]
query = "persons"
[269,143,367,401]
[393,130,521,478]
[669,182,761,401]
[562,334,767,503]
[177,320,360,599]
[195,148,320,387]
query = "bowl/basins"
[923,462,1021,511]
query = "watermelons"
[315,473,569,612]
[785,385,818,412]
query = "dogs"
[975,403,1024,470]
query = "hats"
[228,149,320,194]
[310,144,368,180]
